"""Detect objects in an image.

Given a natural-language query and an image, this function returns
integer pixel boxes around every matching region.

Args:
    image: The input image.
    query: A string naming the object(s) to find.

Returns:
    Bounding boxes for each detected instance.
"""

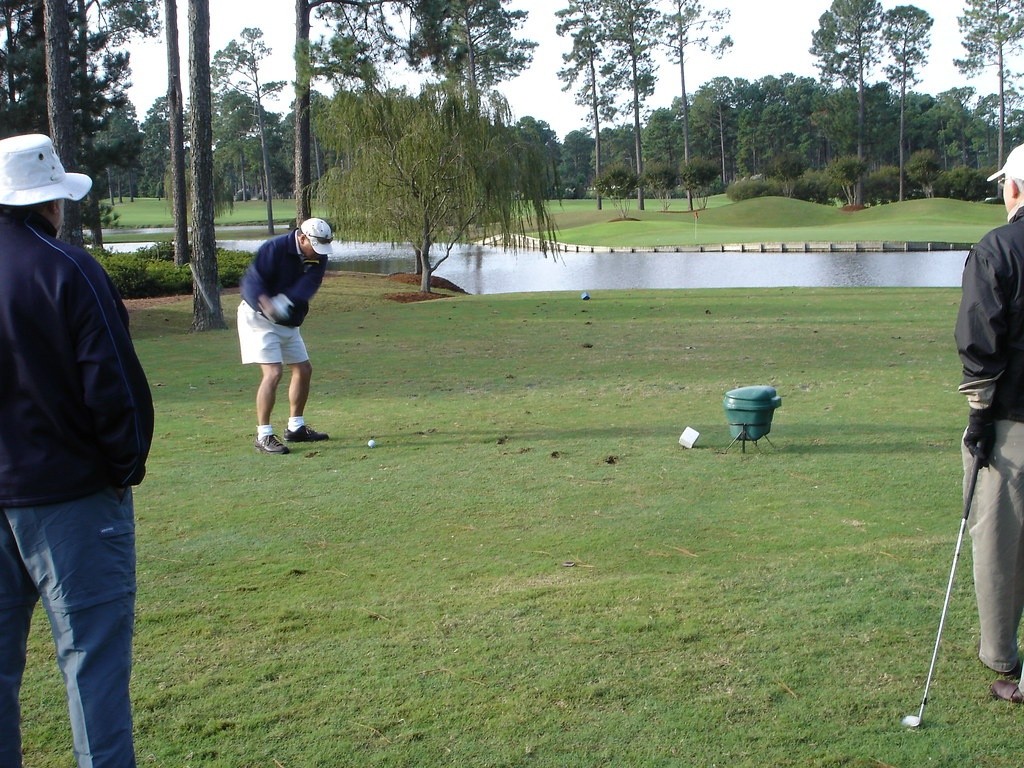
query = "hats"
[301,218,334,255]
[0,134,92,205]
[987,143,1024,182]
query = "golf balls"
[367,440,375,448]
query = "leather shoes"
[992,681,1024,703]
[998,657,1020,678]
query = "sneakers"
[284,425,329,442]
[255,433,289,455]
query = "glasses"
[998,178,1010,186]
[309,235,333,244]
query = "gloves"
[963,407,996,471]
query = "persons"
[236,217,334,452]
[2,129,155,768]
[955,146,1024,704]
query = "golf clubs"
[902,439,985,730]
